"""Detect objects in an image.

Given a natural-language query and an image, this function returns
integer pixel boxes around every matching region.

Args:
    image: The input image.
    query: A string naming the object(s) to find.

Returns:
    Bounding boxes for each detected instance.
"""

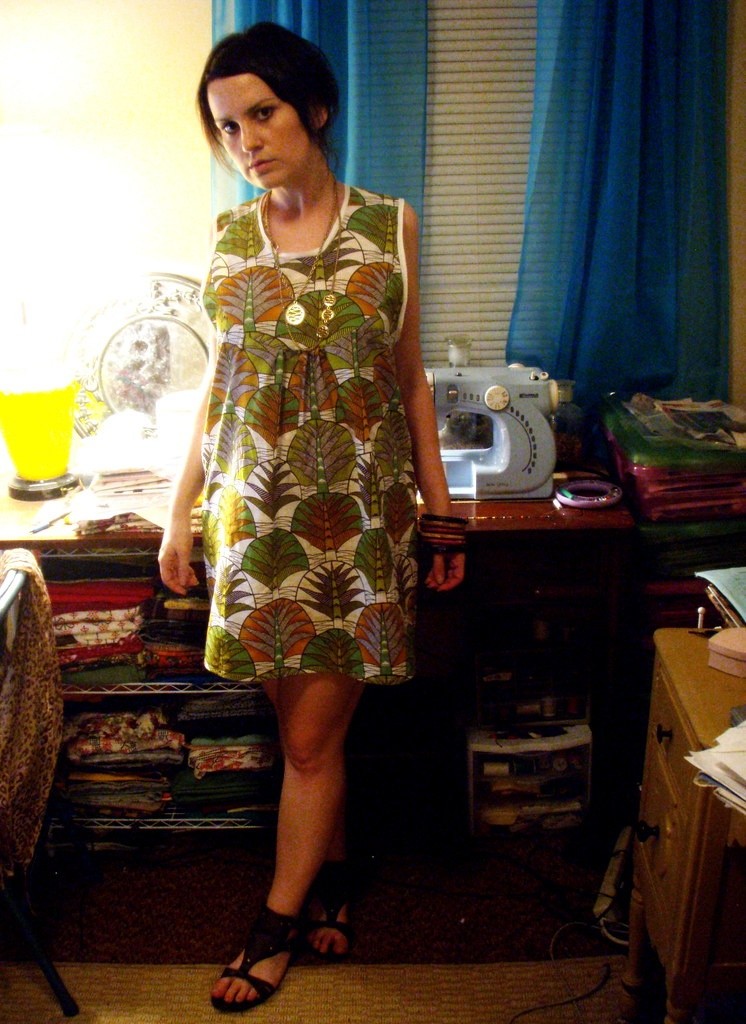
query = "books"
[684,718,746,816]
[72,454,204,535]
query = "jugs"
[0,366,85,501]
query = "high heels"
[300,843,356,962]
[210,905,298,1013]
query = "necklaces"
[263,174,342,351]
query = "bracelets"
[420,513,468,552]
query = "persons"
[158,21,468,1011]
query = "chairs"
[0,549,95,1015]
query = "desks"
[0,491,635,561]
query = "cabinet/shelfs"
[46,674,271,832]
[626,624,745,1024]
